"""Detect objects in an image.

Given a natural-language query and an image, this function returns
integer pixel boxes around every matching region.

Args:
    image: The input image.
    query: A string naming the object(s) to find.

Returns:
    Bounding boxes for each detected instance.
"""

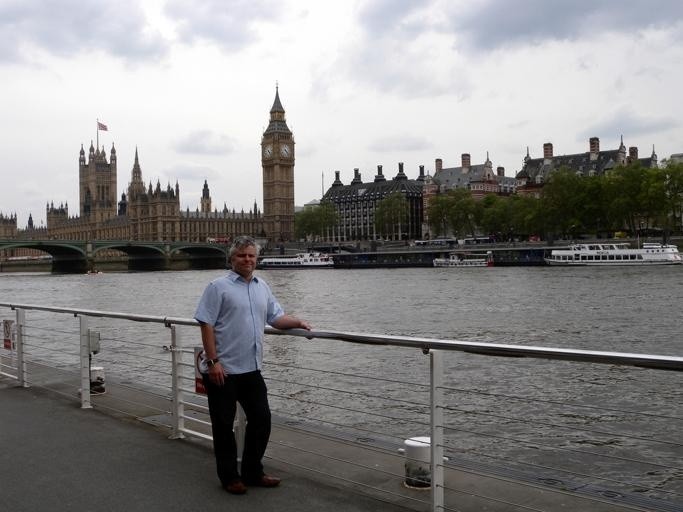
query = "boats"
[432,251,495,268]
[82,270,102,275]
[257,251,336,269]
[541,241,683,267]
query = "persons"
[191,233,315,495]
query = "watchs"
[205,357,219,367]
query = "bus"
[413,237,457,246]
[207,237,231,247]
[464,233,496,244]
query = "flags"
[96,121,107,132]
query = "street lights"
[571,224,576,239]
[641,221,645,243]
[509,225,515,242]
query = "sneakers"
[225,482,248,494]
[255,476,281,487]
